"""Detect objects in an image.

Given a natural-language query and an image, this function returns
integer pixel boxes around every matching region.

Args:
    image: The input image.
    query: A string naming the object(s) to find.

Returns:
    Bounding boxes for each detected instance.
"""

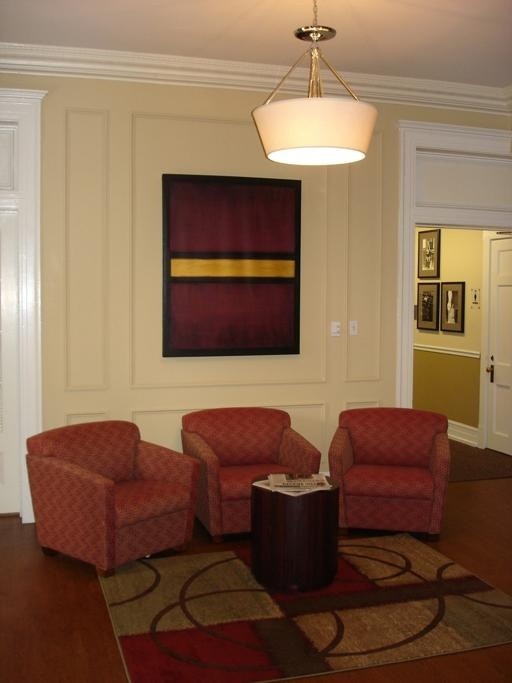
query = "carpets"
[445,447,511,481]
[93,531,511,683]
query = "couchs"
[24,418,204,577]
[178,405,323,543]
[326,405,451,537]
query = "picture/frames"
[159,170,303,357]
[415,280,439,331]
[417,227,441,277]
[439,280,467,332]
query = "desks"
[250,474,340,594]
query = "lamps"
[250,0,380,167]
